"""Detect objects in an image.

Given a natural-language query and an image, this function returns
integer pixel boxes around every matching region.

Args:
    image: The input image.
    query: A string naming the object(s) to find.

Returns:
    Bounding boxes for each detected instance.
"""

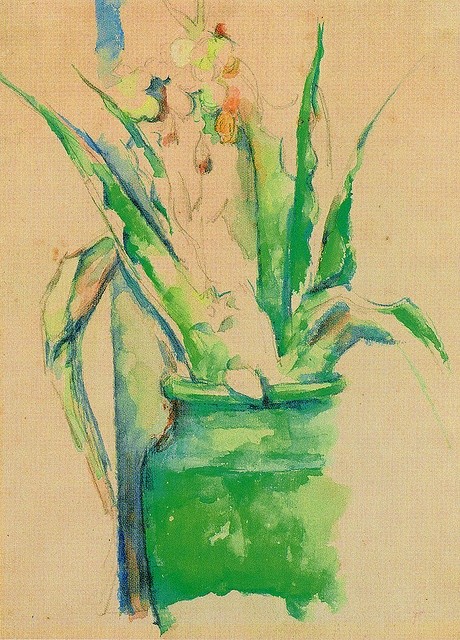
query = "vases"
[138,373,349,636]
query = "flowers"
[1,0,460,623]
